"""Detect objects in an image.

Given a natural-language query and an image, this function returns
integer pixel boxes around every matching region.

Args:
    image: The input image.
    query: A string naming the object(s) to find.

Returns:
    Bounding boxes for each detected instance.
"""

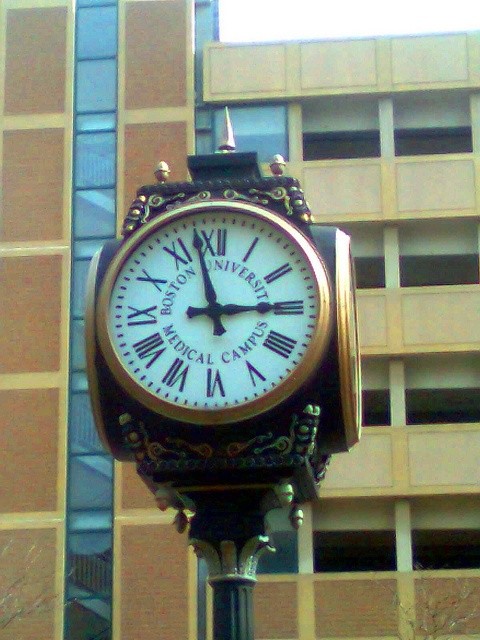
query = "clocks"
[95,190,335,430]
